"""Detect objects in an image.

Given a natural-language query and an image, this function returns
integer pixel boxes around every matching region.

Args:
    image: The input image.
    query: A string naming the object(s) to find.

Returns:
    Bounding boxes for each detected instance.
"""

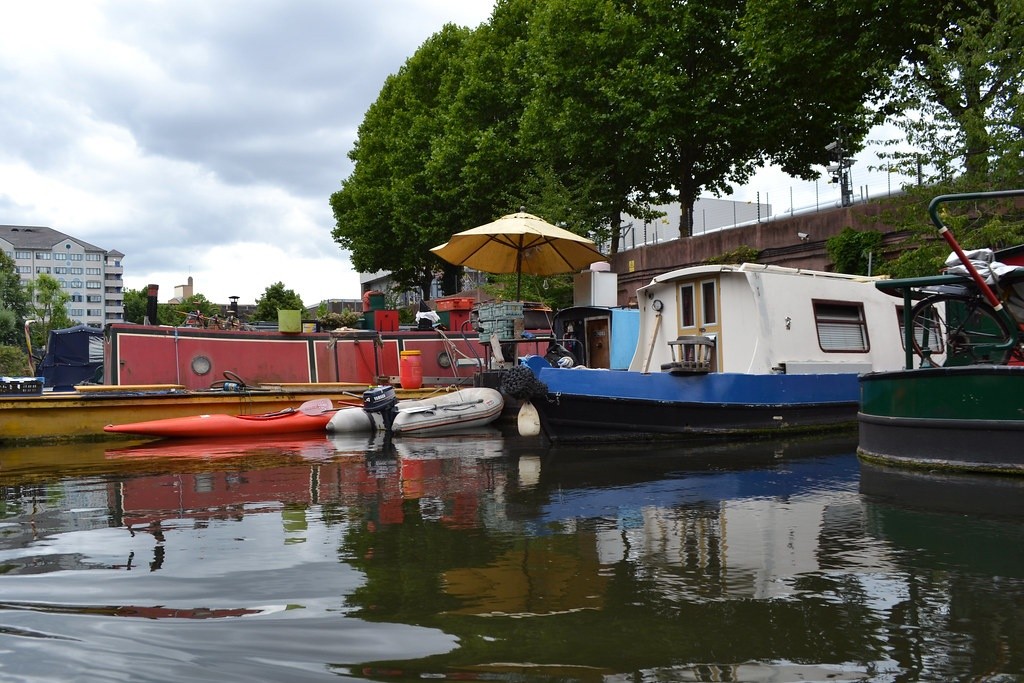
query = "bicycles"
[908,256,1024,367]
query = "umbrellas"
[430,205,613,302]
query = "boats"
[104,406,351,437]
[495,262,946,454]
[326,385,505,433]
[101,298,555,386]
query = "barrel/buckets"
[302,320,320,333]
[399,350,423,389]
[276,307,302,333]
[363,293,384,329]
[375,309,399,331]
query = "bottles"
[223,383,240,391]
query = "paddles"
[404,398,483,414]
[281,398,334,416]
